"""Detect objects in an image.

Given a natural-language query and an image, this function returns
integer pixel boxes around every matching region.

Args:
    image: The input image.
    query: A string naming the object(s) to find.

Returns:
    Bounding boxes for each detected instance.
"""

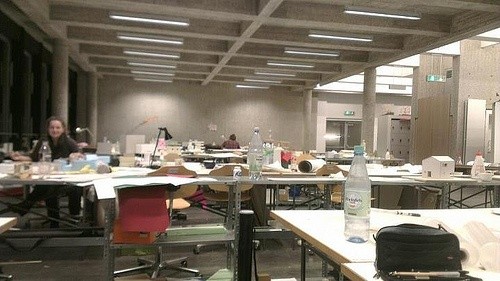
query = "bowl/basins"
[203,161,216,169]
[53,159,66,172]
[479,173,492,182]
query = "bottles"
[343,145,370,244]
[470,152,485,179]
[386,149,390,160]
[248,127,263,182]
[273,143,281,167]
[38,141,52,179]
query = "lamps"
[150,126,173,168]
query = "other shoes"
[6,202,27,217]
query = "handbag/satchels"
[372,222,463,272]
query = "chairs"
[144,166,198,219]
[194,163,259,255]
[314,162,377,204]
[163,152,182,162]
[113,185,201,281]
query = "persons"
[5,115,83,229]
[220,134,241,149]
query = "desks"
[0,143,500,281]
